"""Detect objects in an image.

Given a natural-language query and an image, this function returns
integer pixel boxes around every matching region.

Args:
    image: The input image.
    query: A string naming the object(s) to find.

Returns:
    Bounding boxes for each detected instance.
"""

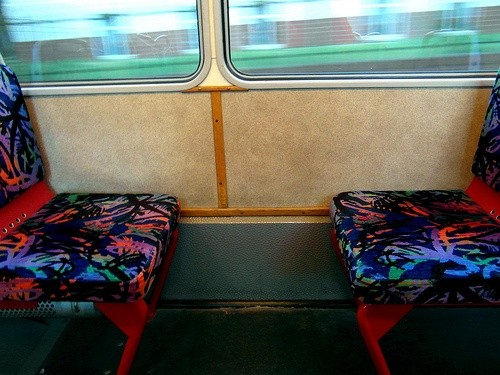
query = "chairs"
[0,55,182,375]
[329,68,500,375]
